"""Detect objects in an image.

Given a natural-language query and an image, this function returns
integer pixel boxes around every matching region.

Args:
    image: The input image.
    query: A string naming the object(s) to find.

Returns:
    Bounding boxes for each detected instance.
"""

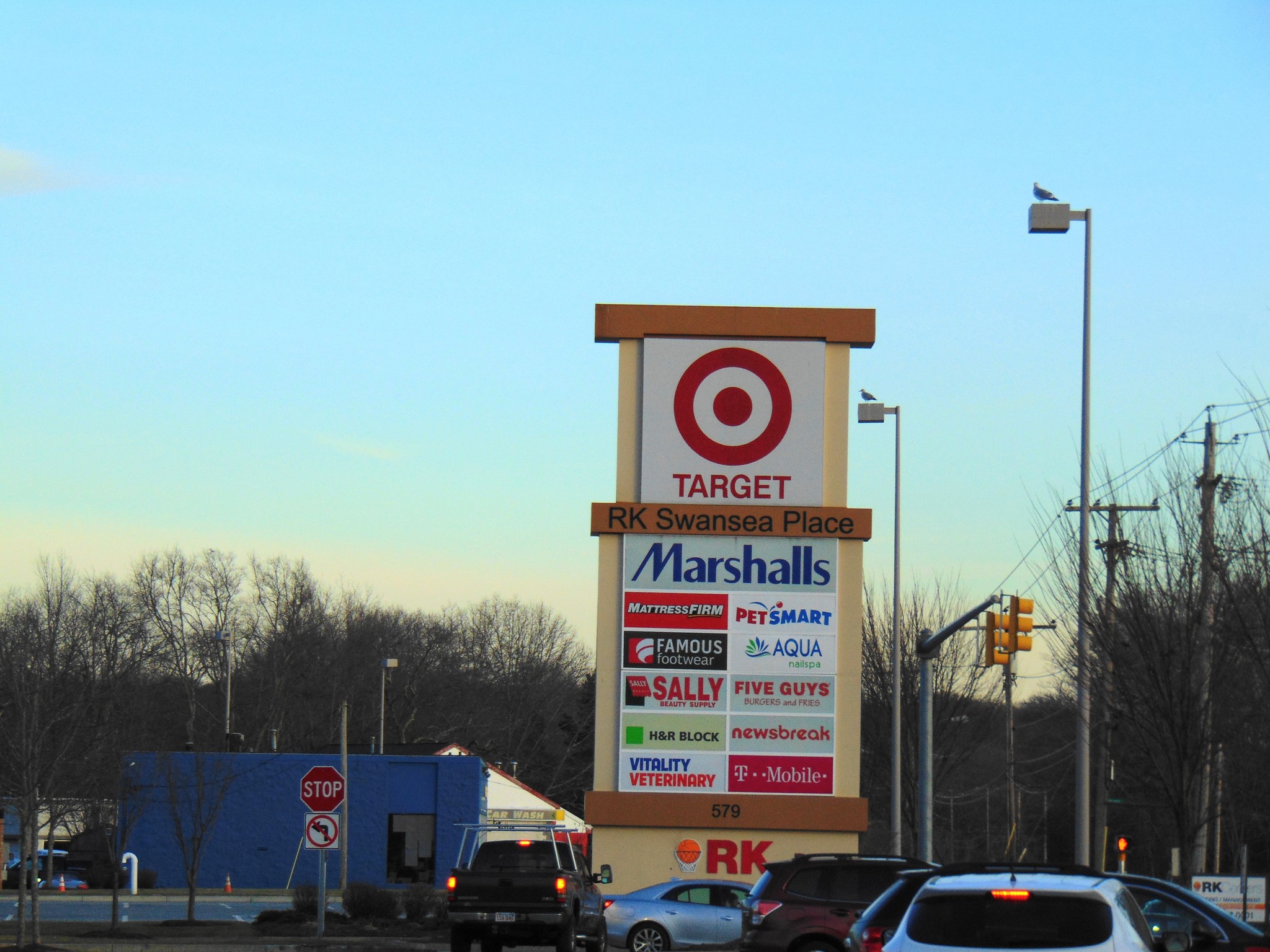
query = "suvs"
[845,866,1269,952]
[8,849,69,890]
[737,852,943,952]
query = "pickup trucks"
[445,819,606,952]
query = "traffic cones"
[224,872,232,892]
[59,873,65,892]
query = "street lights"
[379,657,398,753]
[858,400,900,858]
[216,630,233,752]
[1028,202,1093,871]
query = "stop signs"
[301,766,345,813]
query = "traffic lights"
[1116,835,1129,852]
[1007,597,1033,652]
[985,611,1010,668]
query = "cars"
[602,876,754,952]
